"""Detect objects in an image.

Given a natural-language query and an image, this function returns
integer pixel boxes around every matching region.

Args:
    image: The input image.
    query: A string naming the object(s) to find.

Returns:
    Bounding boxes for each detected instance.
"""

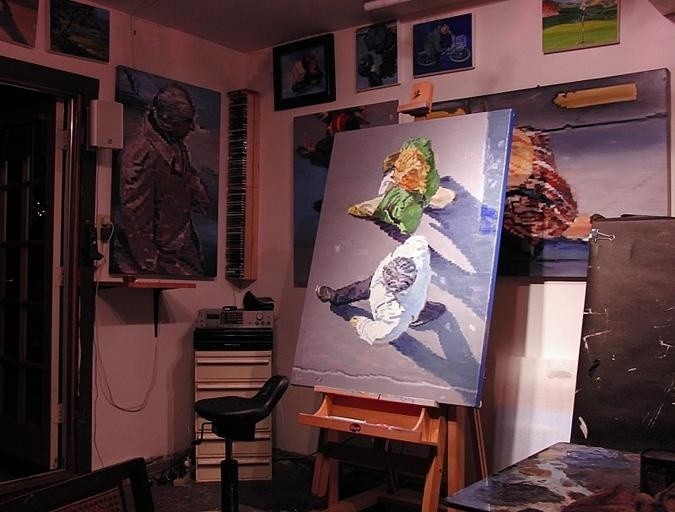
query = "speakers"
[86,100,124,149]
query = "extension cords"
[173,466,193,486]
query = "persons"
[348,134,458,236]
[313,235,447,348]
[117,82,213,277]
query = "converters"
[184,459,192,468]
[101,228,114,240]
[101,217,110,226]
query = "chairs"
[190,373,288,511]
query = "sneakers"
[315,284,334,302]
[408,302,445,327]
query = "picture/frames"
[272,31,337,110]
